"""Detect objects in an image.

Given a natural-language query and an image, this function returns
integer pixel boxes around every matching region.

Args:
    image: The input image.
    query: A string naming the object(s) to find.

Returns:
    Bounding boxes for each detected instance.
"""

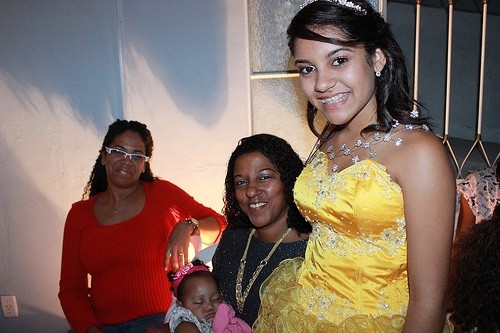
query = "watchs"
[183,217,199,236]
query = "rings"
[178,252,185,256]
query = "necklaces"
[324,118,430,171]
[107,189,136,213]
[236,226,292,315]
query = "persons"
[59,119,228,333]
[252,0,455,333]
[166,259,252,333]
[445,158,500,333]
[210,133,312,325]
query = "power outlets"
[0,295,19,318]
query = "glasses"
[105,146,150,162]
